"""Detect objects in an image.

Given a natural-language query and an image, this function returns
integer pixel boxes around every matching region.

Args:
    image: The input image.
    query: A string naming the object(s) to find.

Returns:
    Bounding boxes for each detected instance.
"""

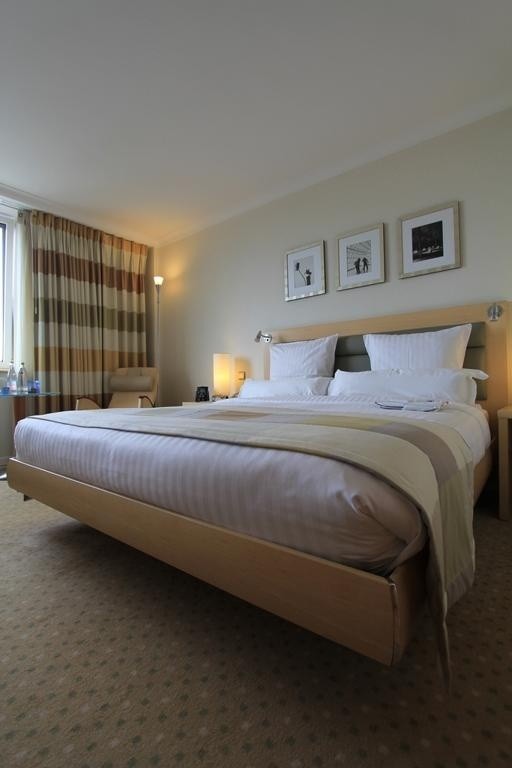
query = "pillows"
[363,323,474,368]
[110,375,153,392]
[239,378,330,396]
[268,334,339,377]
[327,367,488,406]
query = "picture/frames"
[284,239,326,302]
[336,223,385,291]
[397,198,461,278]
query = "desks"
[0,392,61,480]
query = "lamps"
[153,275,164,406]
[213,352,233,401]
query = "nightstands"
[496,405,512,520]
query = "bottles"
[6,361,40,393]
[195,386,209,403]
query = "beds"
[6,300,512,667]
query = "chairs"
[76,367,159,410]
